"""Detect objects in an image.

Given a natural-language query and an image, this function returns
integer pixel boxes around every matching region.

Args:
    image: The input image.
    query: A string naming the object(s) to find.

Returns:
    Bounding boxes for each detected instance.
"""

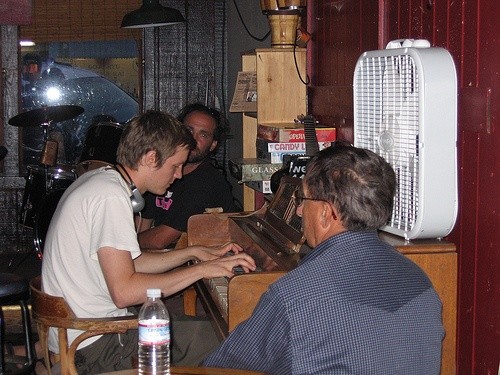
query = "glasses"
[291,190,337,221]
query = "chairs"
[8,169,54,269]
[30,273,142,374]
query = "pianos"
[184,150,457,375]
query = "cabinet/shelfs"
[244,47,306,211]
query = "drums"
[30,197,63,262]
[73,119,127,173]
[14,162,78,232]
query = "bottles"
[137,289,170,375]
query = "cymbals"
[7,105,84,128]
[0,144,9,161]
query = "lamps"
[121,0,185,30]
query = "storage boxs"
[256,122,336,164]
[245,180,273,193]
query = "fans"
[346,37,458,241]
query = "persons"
[40,109,221,375]
[198,145,445,375]
[126,101,233,314]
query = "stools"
[0,271,35,375]
